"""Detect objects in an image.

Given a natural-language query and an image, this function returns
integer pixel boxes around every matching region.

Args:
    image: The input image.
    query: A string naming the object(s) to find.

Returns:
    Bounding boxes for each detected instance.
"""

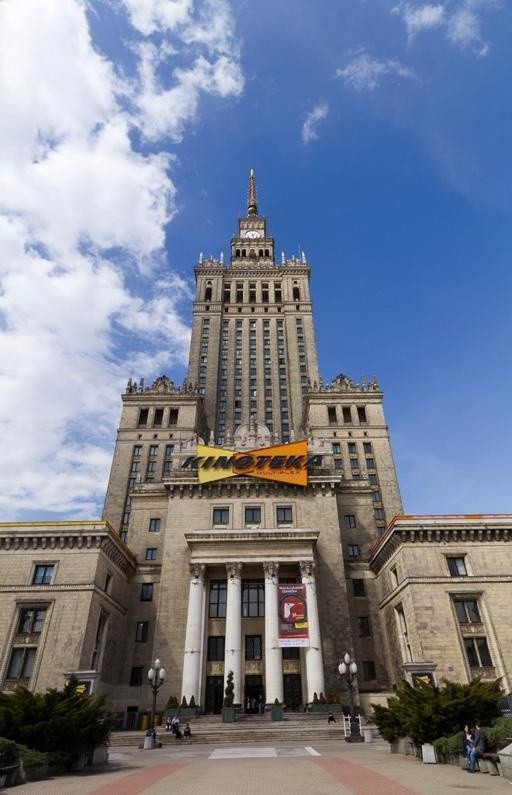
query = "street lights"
[338,651,365,743]
[137,657,167,749]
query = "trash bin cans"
[144,737,154,749]
[223,708,235,723]
[93,747,108,764]
[422,743,438,764]
[272,706,284,720]
[363,728,373,744]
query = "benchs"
[476,738,511,777]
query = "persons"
[463,725,475,769]
[246,695,264,714]
[468,723,485,772]
[166,716,191,739]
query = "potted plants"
[310,690,345,713]
[165,695,202,716]
[220,671,237,723]
[270,697,284,721]
